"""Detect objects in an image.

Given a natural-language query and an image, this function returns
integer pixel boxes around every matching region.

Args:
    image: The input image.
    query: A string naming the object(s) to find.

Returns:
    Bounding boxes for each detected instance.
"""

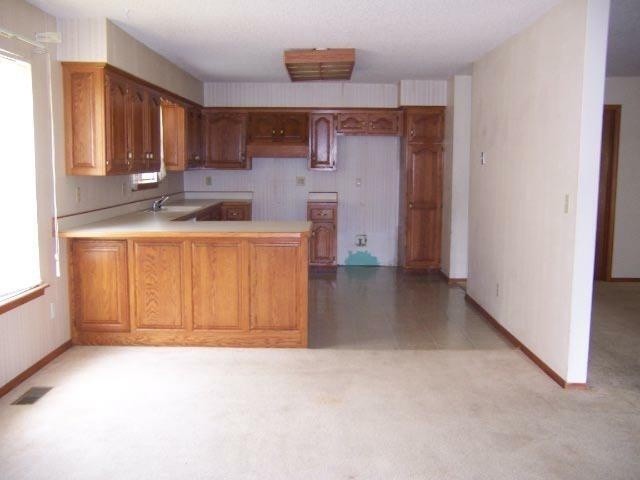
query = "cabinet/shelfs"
[204,113,251,170]
[405,114,442,273]
[335,112,404,133]
[63,66,161,173]
[247,113,309,157]
[309,108,337,171]
[222,203,248,222]
[179,204,222,221]
[63,235,309,349]
[309,201,335,265]
[163,102,203,170]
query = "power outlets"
[295,175,305,187]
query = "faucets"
[153,196,169,212]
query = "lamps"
[283,50,355,81]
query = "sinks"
[160,205,200,213]
